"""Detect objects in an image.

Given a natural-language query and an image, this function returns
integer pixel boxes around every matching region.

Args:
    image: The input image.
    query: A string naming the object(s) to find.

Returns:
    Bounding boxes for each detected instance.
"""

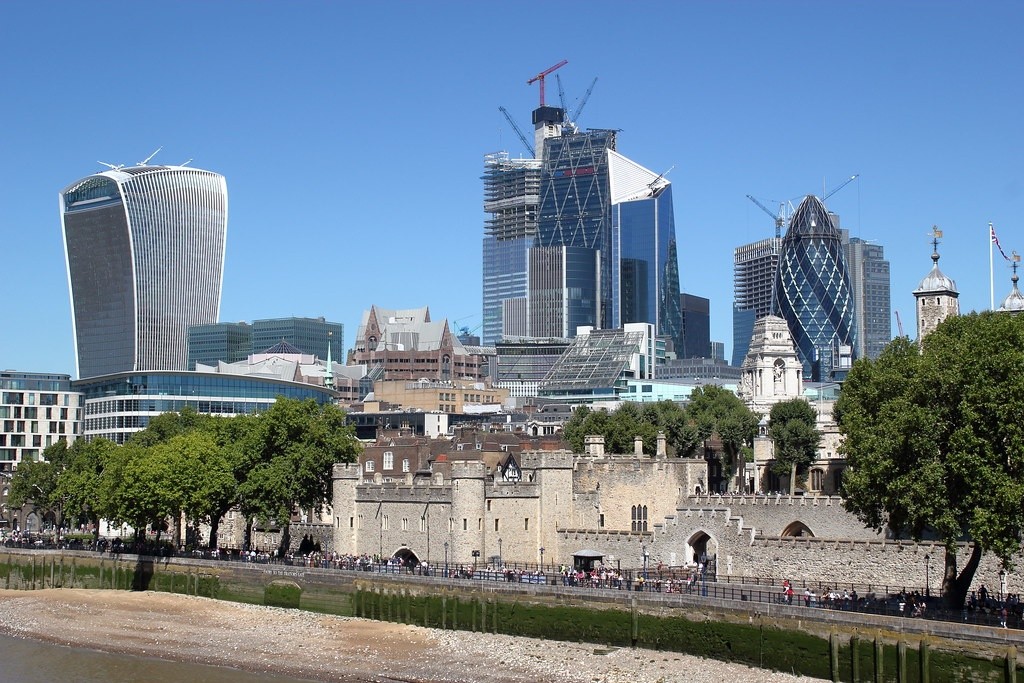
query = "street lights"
[923,554,930,597]
[323,539,331,569]
[498,538,503,566]
[645,550,650,580]
[444,542,449,563]
[998,570,1005,602]
[123,527,127,538]
[265,539,270,555]
[540,548,545,570]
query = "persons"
[176,543,435,577]
[447,552,708,594]
[780,579,1024,630]
[697,490,781,496]
[0,524,174,557]
